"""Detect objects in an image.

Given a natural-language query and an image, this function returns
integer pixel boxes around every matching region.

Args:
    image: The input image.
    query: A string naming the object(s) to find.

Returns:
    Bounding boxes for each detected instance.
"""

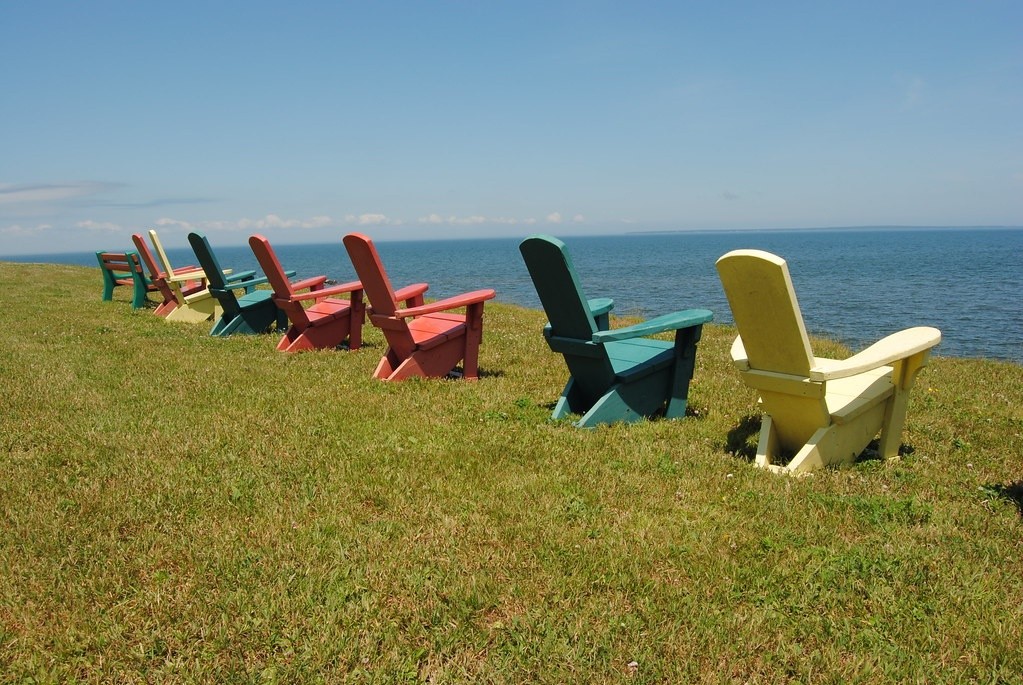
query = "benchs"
[96,250,181,309]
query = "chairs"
[714,248,941,478]
[130,230,495,383]
[520,235,714,428]
